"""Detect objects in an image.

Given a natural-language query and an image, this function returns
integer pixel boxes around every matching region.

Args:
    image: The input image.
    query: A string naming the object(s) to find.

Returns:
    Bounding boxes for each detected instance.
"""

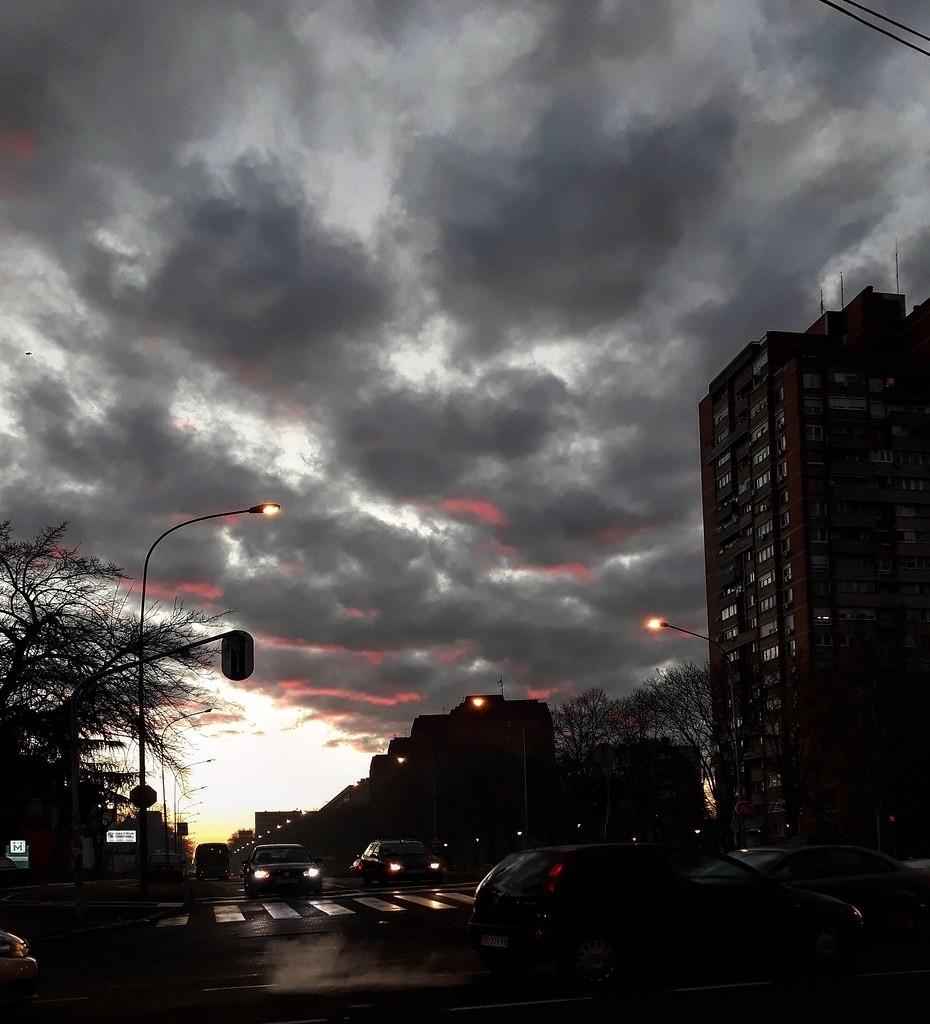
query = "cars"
[0,929,39,1006]
[146,853,186,883]
[468,844,929,997]
[242,843,323,898]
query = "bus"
[195,843,233,883]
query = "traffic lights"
[888,812,897,833]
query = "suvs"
[361,834,442,887]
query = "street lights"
[472,697,528,852]
[154,709,212,862]
[170,760,214,853]
[643,618,745,851]
[139,502,280,902]
